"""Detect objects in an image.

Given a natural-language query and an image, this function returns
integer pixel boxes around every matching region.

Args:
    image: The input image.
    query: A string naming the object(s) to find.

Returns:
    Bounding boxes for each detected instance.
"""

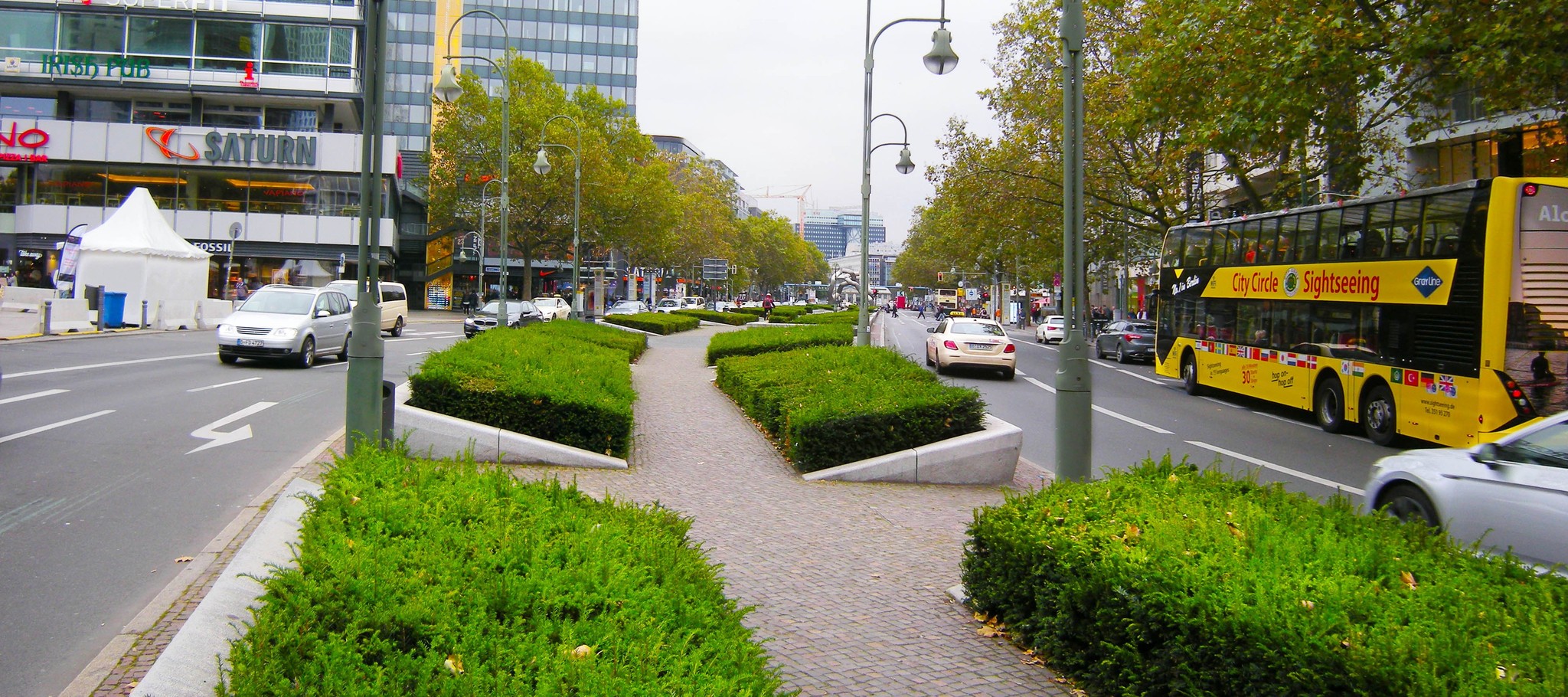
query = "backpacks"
[983,310,987,314]
[765,298,770,306]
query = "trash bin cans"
[85,283,99,325]
[382,380,394,450]
[103,292,126,329]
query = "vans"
[324,278,409,337]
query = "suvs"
[216,282,353,368]
[653,297,688,314]
[680,296,706,310]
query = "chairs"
[55,195,66,205]
[1337,328,1357,345]
[1172,233,1461,269]
[109,203,118,206]
[162,198,172,209]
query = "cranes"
[749,189,812,239]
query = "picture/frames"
[272,269,289,285]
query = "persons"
[917,304,925,320]
[1030,306,1041,323]
[883,302,899,318]
[1083,304,1117,343]
[728,293,731,302]
[532,287,573,308]
[645,295,652,309]
[235,277,248,300]
[782,298,788,302]
[762,293,776,320]
[1126,306,1152,321]
[835,295,846,310]
[935,304,945,321]
[637,296,640,301]
[460,289,499,315]
[7,270,52,288]
[1017,307,1026,330]
[958,306,1001,323]
[755,293,760,302]
[853,301,859,306]
[906,299,915,310]
[1530,351,1552,381]
[506,288,521,300]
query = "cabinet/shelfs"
[222,271,240,292]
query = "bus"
[931,288,959,318]
[1152,171,1568,447]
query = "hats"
[766,293,772,296]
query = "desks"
[250,209,260,212]
[67,197,81,206]
[107,198,120,207]
[154,199,160,208]
[208,206,221,211]
[285,209,300,214]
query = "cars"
[463,298,544,339]
[925,310,1016,379]
[1034,314,1074,345]
[707,298,819,310]
[1362,406,1568,580]
[1095,319,1170,364]
[529,296,571,322]
[604,299,649,317]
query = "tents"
[56,185,214,324]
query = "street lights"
[431,7,512,329]
[458,178,511,315]
[533,115,581,322]
[856,0,960,348]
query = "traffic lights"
[937,272,943,282]
